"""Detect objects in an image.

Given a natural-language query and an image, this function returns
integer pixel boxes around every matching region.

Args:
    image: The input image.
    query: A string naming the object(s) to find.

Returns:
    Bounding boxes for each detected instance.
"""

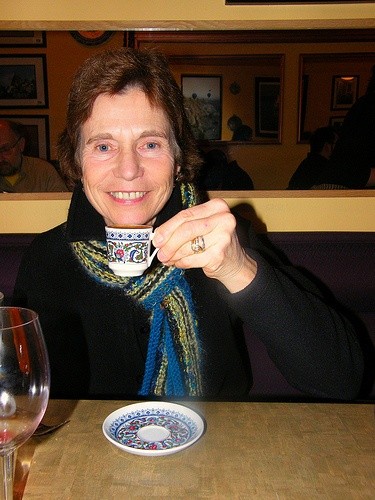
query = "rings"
[191,235,204,253]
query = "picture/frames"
[254,74,306,139]
[0,54,49,109]
[328,115,346,127]
[0,114,49,161]
[180,74,222,140]
[331,74,360,110]
[0,30,47,47]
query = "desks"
[1,394,375,500]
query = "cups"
[106,225,159,278]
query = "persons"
[328,64,375,190]
[193,148,255,190]
[285,126,343,189]
[12,46,369,405]
[227,115,253,141]
[0,118,70,192]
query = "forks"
[33,420,71,436]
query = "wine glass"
[0,308,51,500]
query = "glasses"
[0,136,21,156]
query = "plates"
[103,401,204,456]
